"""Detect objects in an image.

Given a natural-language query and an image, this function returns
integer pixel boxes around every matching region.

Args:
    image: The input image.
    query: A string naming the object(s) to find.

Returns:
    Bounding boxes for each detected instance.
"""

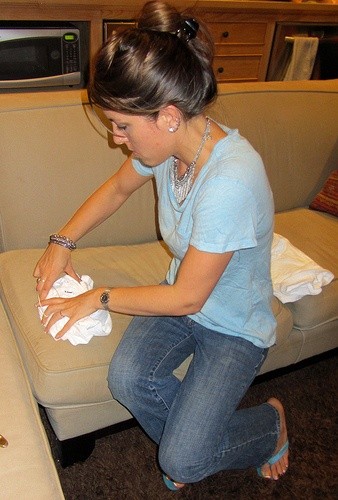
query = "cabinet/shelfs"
[1,0,338,80]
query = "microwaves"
[0,22,82,89]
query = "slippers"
[256,398,289,479]
[160,471,179,491]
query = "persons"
[32,0,291,491]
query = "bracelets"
[48,234,76,251]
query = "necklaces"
[173,117,211,202]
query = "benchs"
[0,79,338,500]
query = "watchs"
[100,287,112,310]
